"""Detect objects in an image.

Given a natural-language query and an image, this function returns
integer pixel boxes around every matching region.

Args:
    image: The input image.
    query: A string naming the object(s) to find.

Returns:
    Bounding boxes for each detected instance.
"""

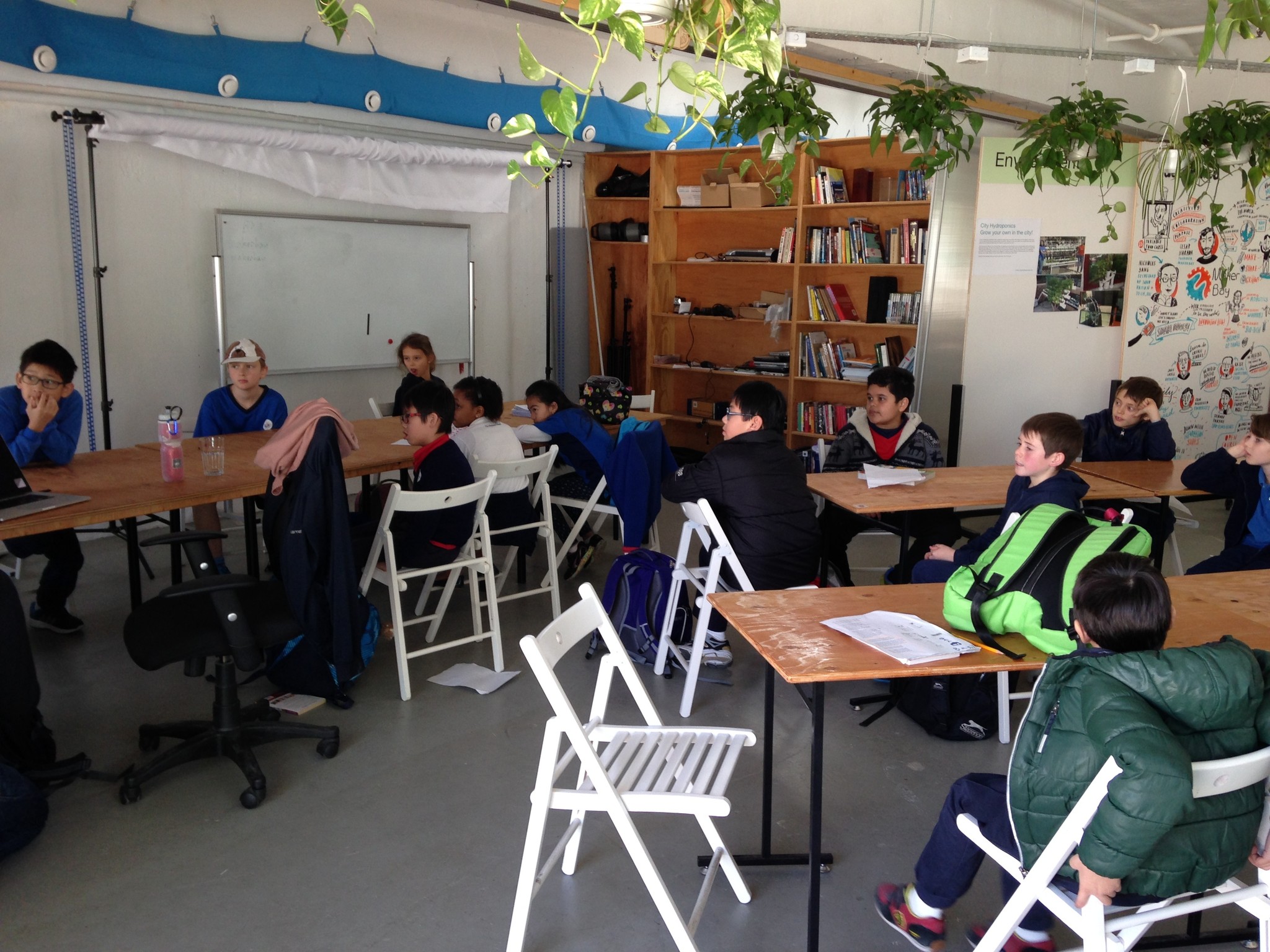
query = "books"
[776,217,797,264]
[799,330,916,382]
[796,401,867,436]
[805,217,928,265]
[809,157,849,204]
[897,169,933,202]
[807,282,861,323]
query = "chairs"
[541,418,679,589]
[817,438,910,563]
[653,498,818,719]
[955,742,1270,952]
[414,443,561,643]
[356,467,504,701]
[998,509,1134,744]
[506,582,758,952]
[118,396,360,810]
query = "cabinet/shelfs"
[582,134,937,452]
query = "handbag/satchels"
[887,670,1019,740]
[578,377,633,424]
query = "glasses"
[22,373,65,389]
[400,413,430,424]
[727,407,763,430]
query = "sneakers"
[29,601,84,632]
[964,924,1055,952]
[675,632,733,667]
[875,881,946,952]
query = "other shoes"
[563,540,595,582]
[586,536,606,570]
[213,564,231,575]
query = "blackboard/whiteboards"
[199,208,470,376]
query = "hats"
[220,339,266,364]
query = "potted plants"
[1106,120,1204,220]
[500,0,784,191]
[863,58,985,181]
[1178,98,1270,288]
[709,63,839,206]
[1013,80,1149,196]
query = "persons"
[1080,376,1177,565]
[0,339,85,634]
[423,375,535,589]
[510,378,617,582]
[354,376,476,574]
[192,338,289,576]
[874,550,1270,952]
[910,412,1090,584]
[816,367,947,589]
[392,333,445,416]
[659,380,825,667]
[1180,415,1270,576]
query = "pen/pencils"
[950,632,1005,655]
[367,314,369,334]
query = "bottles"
[158,405,184,482]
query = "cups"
[199,436,225,476]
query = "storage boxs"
[737,290,785,320]
[727,172,781,208]
[700,167,746,206]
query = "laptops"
[0,435,90,524]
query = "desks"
[705,568,1270,952]
[134,418,417,577]
[805,464,1156,588]
[1068,459,1228,570]
[374,398,674,586]
[0,445,267,579]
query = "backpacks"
[599,549,693,672]
[267,591,381,706]
[943,503,1154,657]
